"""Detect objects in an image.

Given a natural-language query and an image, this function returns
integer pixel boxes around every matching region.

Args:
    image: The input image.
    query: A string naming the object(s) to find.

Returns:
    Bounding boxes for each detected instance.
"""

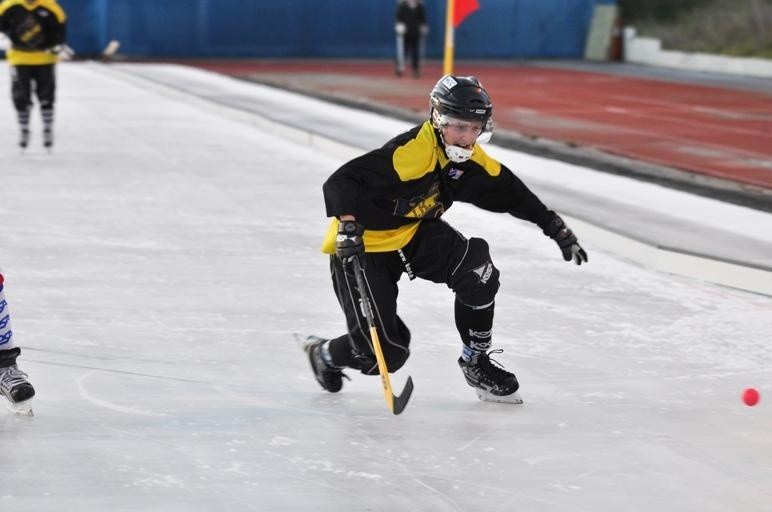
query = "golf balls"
[744,388,760,407]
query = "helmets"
[429,74,493,122]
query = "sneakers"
[17,129,54,148]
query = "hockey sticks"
[353,257,414,415]
[9,40,120,63]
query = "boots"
[302,334,346,394]
[457,350,521,397]
[0,347,36,404]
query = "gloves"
[335,219,370,270]
[541,212,590,268]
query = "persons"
[1,269,35,403]
[396,0,429,79]
[1,0,69,151]
[304,75,589,399]
